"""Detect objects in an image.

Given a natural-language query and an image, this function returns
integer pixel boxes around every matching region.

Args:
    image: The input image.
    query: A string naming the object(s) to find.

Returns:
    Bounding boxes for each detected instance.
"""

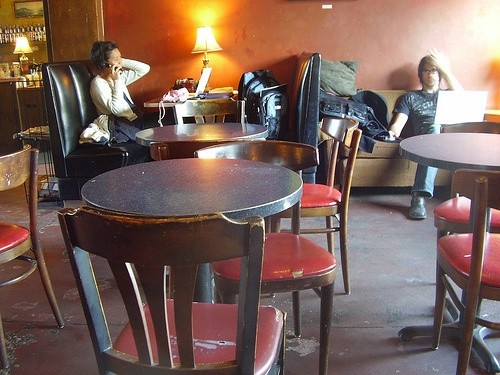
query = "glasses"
[422,69,438,74]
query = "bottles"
[29,63,43,88]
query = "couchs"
[342,90,451,187]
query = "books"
[204,87,233,99]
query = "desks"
[398,133,500,375]
[81,157,303,337]
[134,123,269,161]
[0,79,49,154]
[144,92,238,124]
[12,80,62,206]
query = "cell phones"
[102,64,123,74]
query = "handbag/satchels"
[320,91,368,124]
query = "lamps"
[191,27,223,75]
[13,36,34,61]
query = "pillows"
[320,61,359,96]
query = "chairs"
[269,117,362,298]
[431,122,500,375]
[175,98,245,124]
[57,204,287,375]
[0,143,64,375]
[193,140,336,375]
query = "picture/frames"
[14,0,44,18]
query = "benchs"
[286,51,321,184]
[42,60,175,200]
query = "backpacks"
[237,68,290,140]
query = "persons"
[90,41,175,144]
[385,48,464,221]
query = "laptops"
[422,90,488,127]
[188,68,212,98]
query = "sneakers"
[409,195,427,219]
